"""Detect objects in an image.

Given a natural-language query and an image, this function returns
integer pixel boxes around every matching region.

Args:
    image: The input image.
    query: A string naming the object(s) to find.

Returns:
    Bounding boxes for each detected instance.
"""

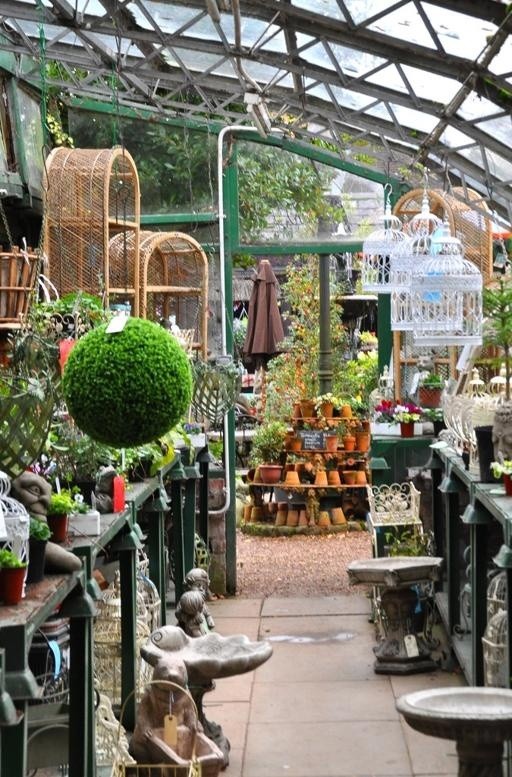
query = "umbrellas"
[242,260,285,421]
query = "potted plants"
[0,485,75,606]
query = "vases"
[473,424,503,482]
[400,421,414,437]
[242,400,369,525]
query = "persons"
[176,570,215,721]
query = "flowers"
[374,398,424,427]
[311,391,338,410]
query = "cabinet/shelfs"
[422,438,512,686]
[239,416,370,536]
[0,445,220,776]
